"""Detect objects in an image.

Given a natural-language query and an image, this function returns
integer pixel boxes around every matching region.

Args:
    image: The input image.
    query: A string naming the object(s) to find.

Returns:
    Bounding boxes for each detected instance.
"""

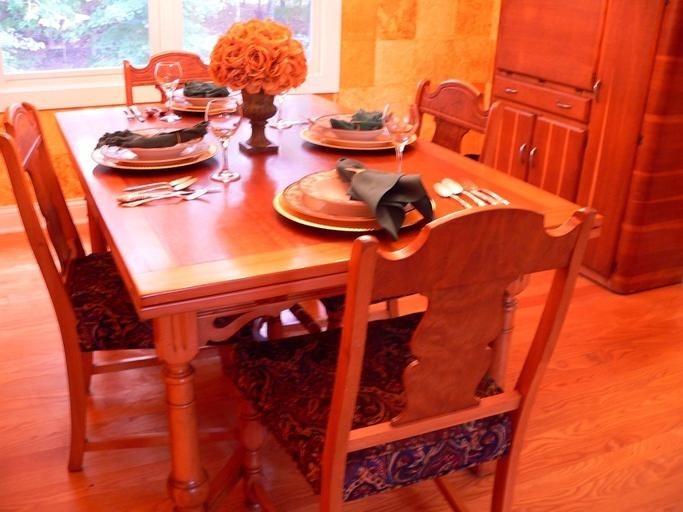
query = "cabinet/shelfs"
[476,0,683,296]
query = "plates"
[269,167,437,240]
[165,85,243,117]
[301,113,417,154]
[91,125,219,170]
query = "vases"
[240,87,279,156]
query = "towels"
[336,152,435,243]
[91,120,210,150]
[182,80,229,98]
[329,107,385,129]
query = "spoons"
[123,104,181,124]
[116,175,209,210]
[433,177,512,214]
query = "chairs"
[222,204,596,511]
[1,99,256,473]
[122,51,216,105]
[408,76,502,167]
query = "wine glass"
[206,98,241,186]
[270,87,295,129]
[382,102,418,175]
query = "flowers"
[209,17,308,93]
